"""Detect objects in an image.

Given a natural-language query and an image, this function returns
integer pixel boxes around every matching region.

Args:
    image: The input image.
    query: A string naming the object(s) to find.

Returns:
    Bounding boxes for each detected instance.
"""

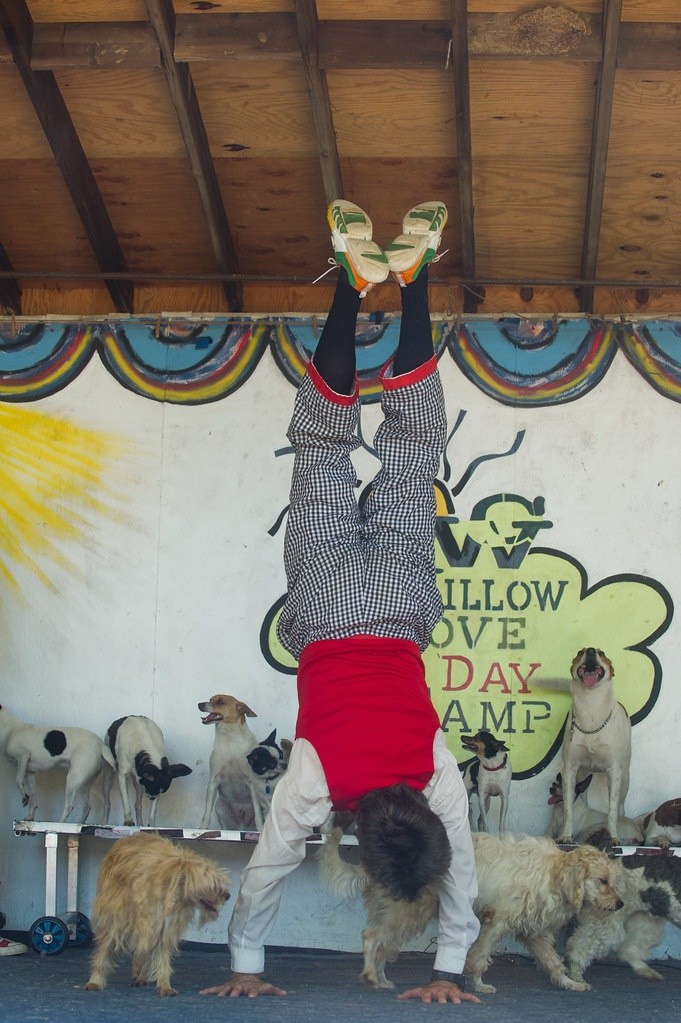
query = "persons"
[199,199,483,1004]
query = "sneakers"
[312,198,389,299]
[385,200,450,287]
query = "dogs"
[546,646,681,986]
[318,826,624,993]
[101,715,193,826]
[460,732,513,835]
[0,705,117,826]
[82,832,231,997]
[246,728,294,825]
[198,694,268,831]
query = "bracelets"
[432,970,466,988]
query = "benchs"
[10,817,681,957]
